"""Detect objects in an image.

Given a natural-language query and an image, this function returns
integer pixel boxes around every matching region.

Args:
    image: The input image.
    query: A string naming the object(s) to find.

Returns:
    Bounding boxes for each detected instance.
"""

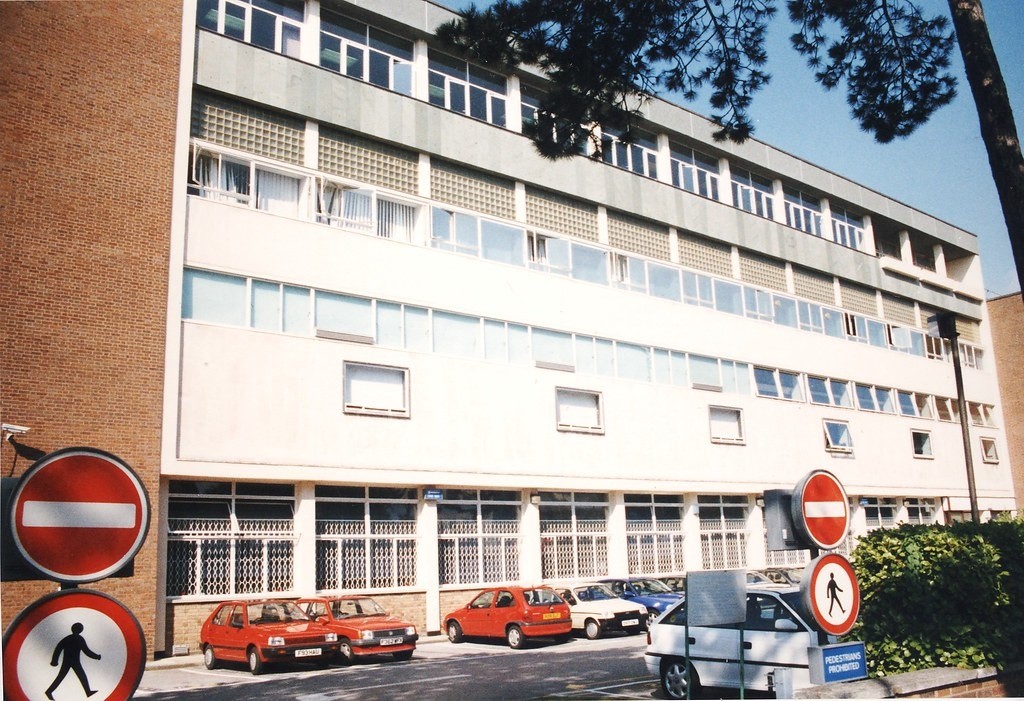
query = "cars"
[746,567,805,587]
[544,582,649,640]
[200,600,341,677]
[576,576,682,634]
[655,575,686,593]
[443,585,572,649]
[643,582,838,699]
[289,594,418,665]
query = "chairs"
[736,601,763,629]
[230,613,242,627]
[497,595,510,607]
[262,606,280,620]
[333,610,342,618]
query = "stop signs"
[6,445,151,586]
[792,468,850,550]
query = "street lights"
[925,308,981,525]
